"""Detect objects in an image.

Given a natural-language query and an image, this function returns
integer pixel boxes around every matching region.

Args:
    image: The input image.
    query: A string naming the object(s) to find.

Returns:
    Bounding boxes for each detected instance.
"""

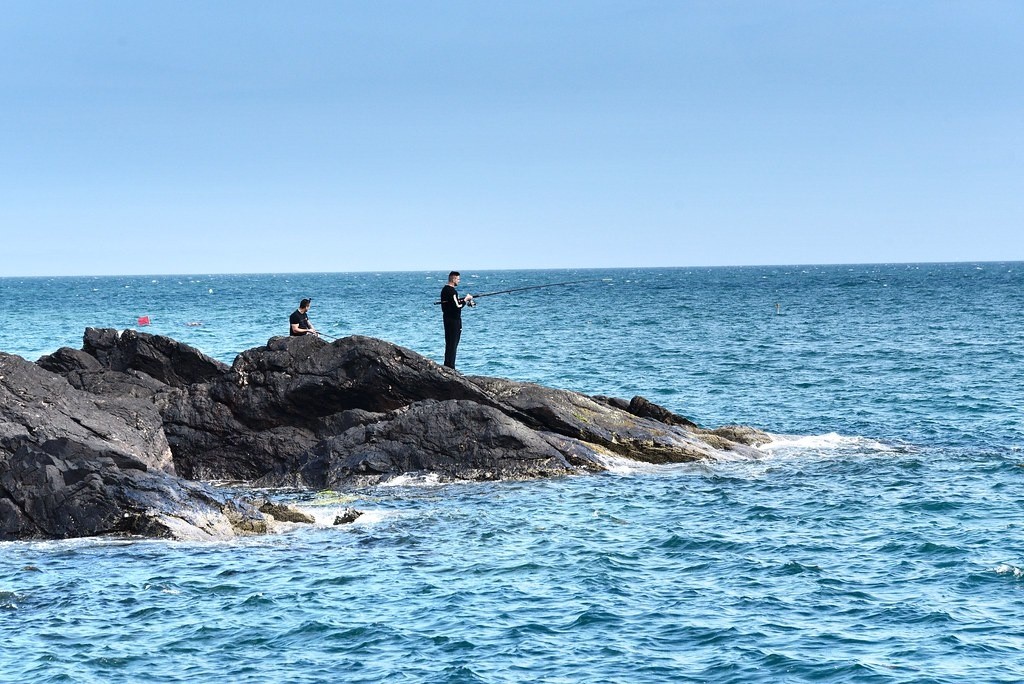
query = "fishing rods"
[314,331,339,340]
[432,278,615,306]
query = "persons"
[441,271,472,370]
[290,299,316,336]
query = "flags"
[138,316,149,325]
